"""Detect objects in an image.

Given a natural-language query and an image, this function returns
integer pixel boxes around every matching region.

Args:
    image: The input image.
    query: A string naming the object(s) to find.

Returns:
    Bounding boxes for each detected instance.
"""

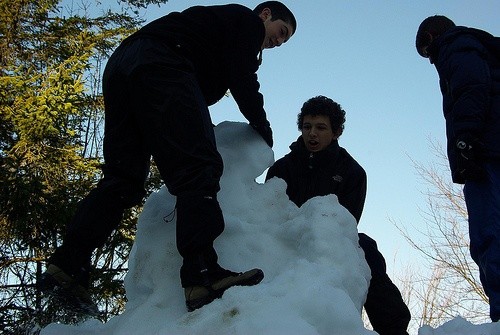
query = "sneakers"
[185,268,264,313]
[42,264,101,317]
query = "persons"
[263,92,412,335]
[38,0,298,312]
[414,15,500,323]
